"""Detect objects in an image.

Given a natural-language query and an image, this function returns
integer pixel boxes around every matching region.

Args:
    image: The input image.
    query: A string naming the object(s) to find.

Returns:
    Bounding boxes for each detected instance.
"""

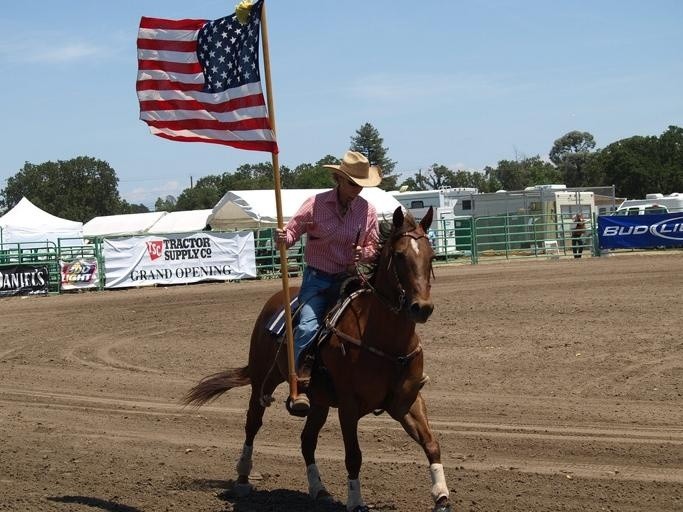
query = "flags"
[136,1,280,156]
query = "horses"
[179,206,453,512]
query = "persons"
[427,227,435,247]
[271,150,384,418]
[571,212,584,258]
[265,236,276,265]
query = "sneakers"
[291,392,310,419]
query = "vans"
[610,204,668,216]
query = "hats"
[322,149,383,187]
